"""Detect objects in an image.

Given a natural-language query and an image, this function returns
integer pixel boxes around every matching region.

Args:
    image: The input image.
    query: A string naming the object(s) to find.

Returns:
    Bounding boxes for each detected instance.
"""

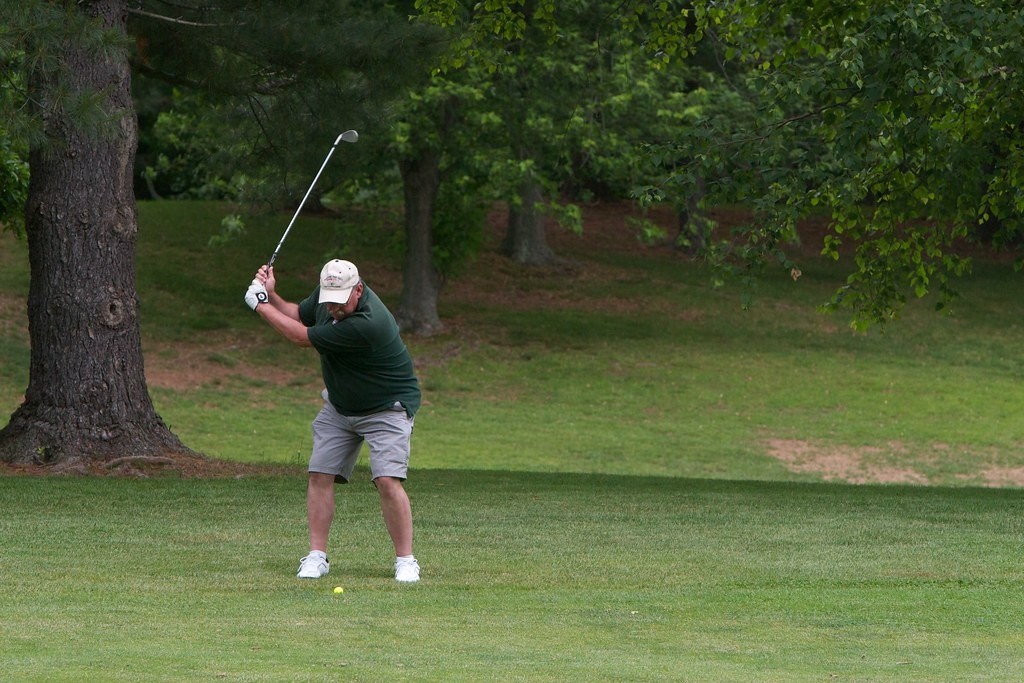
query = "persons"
[244,259,422,581]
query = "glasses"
[322,286,356,307]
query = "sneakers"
[394,556,421,582]
[296,553,329,578]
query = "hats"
[318,258,360,303]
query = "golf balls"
[334,587,343,594]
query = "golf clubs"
[267,129,359,265]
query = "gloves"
[244,279,269,311]
